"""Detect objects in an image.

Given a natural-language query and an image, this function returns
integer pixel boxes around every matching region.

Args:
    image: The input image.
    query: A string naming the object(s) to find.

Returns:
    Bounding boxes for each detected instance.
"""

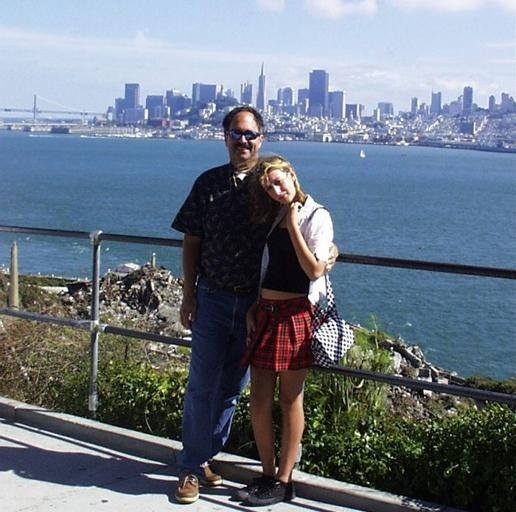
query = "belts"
[222,284,257,296]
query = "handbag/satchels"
[312,310,353,368]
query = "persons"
[238,158,333,507]
[174,107,338,502]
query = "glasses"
[225,128,261,140]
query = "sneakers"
[232,475,296,506]
[174,473,200,503]
[197,462,223,484]
